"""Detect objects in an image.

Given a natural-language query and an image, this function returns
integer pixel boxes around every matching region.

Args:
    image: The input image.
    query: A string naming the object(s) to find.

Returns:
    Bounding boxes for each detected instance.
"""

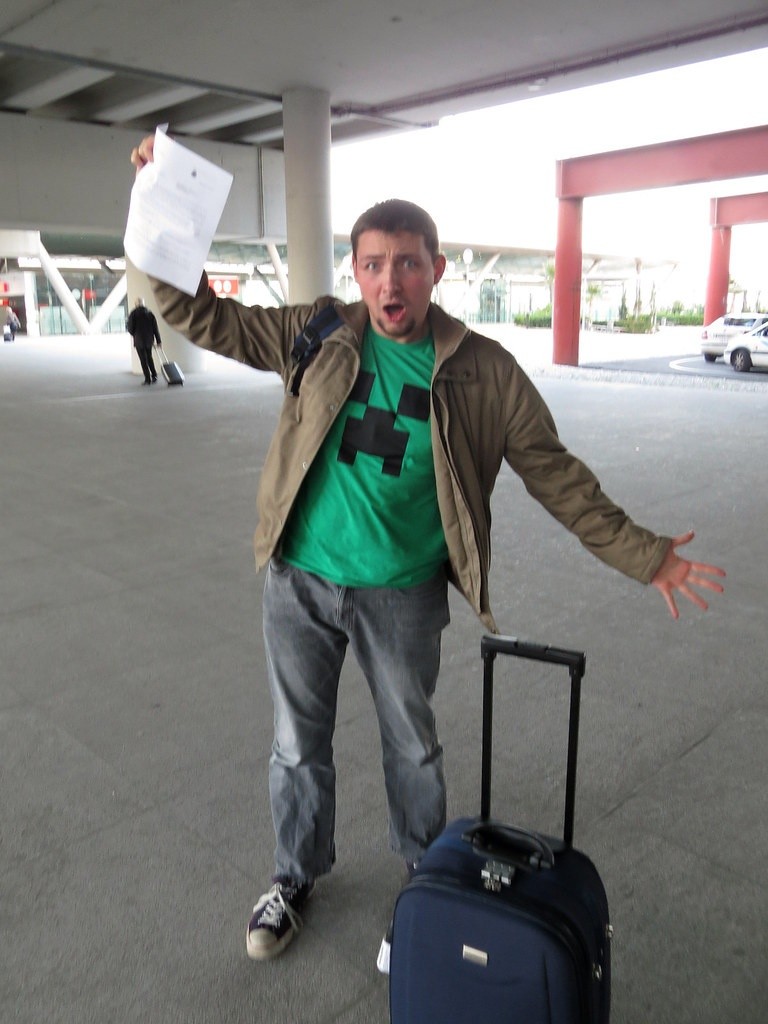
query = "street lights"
[463,248,473,328]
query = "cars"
[699,313,768,363]
[723,322,768,373]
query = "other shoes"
[152,376,157,382]
[141,381,150,385]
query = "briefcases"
[4,333,12,341]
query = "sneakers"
[245,878,317,961]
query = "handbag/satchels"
[3,325,11,334]
[11,320,19,331]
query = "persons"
[131,135,726,960]
[126,298,161,385]
[6,306,21,340]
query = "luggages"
[153,344,184,387]
[387,635,613,1024]
